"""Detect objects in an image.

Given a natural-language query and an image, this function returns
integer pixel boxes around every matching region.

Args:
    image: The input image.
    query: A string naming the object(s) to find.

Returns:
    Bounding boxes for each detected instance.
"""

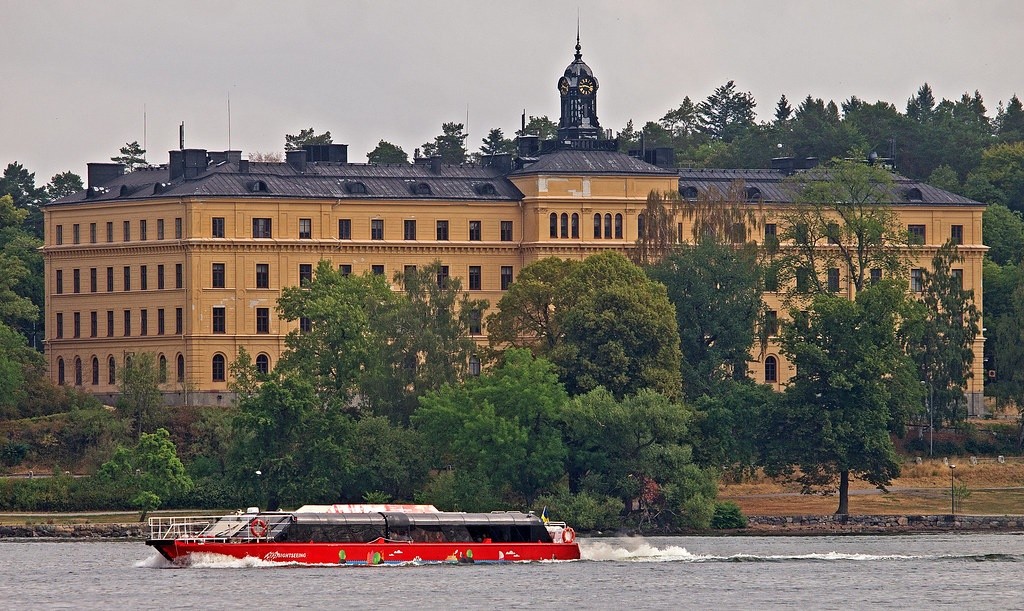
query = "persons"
[388,526,473,545]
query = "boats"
[144,503,581,568]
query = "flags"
[542,509,551,527]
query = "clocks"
[560,79,570,96]
[578,78,594,95]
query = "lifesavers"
[562,528,575,543]
[251,519,265,538]
[198,534,214,538]
